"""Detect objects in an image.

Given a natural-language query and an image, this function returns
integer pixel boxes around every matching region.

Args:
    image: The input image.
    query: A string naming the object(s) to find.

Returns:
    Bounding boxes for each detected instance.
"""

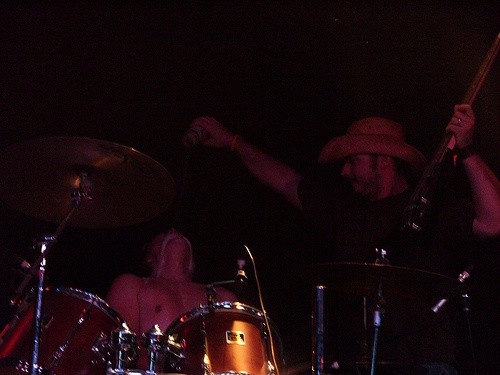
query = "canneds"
[181,125,206,148]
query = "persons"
[190,101,500,375]
[106,226,240,372]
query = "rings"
[458,117,462,125]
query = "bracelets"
[456,143,478,161]
[231,133,239,153]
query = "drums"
[157,299,286,375]
[0,287,140,375]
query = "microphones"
[237,249,248,282]
[432,264,476,313]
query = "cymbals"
[344,359,406,367]
[0,136,178,229]
[287,261,464,299]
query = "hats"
[317,118,426,171]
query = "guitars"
[370,30,500,263]
